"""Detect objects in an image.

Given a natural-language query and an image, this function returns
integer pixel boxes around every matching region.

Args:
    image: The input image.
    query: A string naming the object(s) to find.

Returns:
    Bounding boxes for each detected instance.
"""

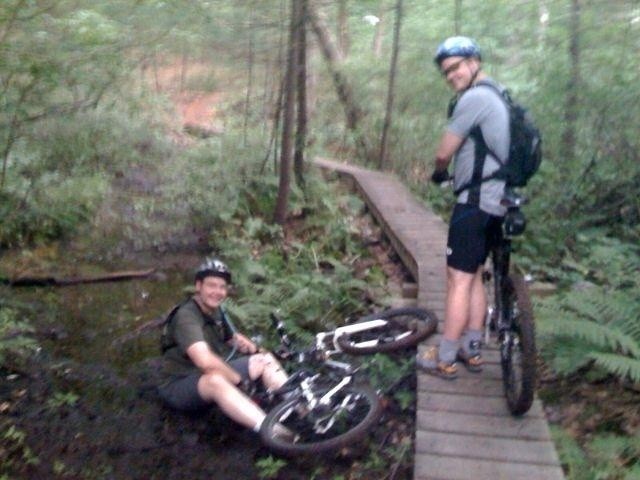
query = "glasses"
[443,57,466,76]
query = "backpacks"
[475,82,544,188]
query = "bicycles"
[445,175,537,415]
[259,308,439,455]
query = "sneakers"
[416,347,458,380]
[457,343,484,372]
[273,429,300,446]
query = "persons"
[154,259,308,447]
[412,34,514,384]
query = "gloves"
[431,168,450,185]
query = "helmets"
[194,259,232,284]
[435,36,481,65]
